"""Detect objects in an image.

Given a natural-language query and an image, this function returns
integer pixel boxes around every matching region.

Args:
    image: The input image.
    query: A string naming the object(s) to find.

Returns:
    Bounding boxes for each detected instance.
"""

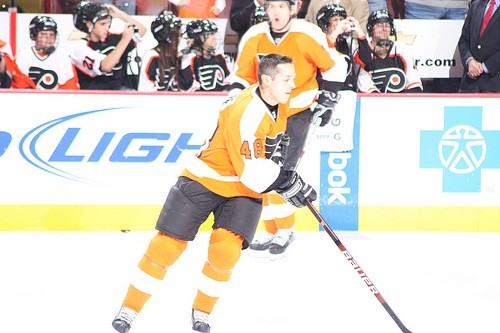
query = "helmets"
[316,4,347,32]
[150,13,183,46]
[72,1,109,32]
[185,18,218,38]
[366,10,395,37]
[28,16,57,40]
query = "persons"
[0,53,11,88]
[226,0,347,260]
[306,0,424,93]
[138,0,233,93]
[6,16,80,90]
[405,0,500,93]
[111,53,338,333]
[66,0,147,91]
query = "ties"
[480,0,496,35]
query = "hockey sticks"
[305,199,415,333]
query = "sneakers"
[189,309,211,333]
[111,305,140,333]
[249,235,274,258]
[268,227,297,261]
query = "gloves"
[219,88,242,110]
[270,134,290,166]
[309,90,341,128]
[272,169,317,209]
